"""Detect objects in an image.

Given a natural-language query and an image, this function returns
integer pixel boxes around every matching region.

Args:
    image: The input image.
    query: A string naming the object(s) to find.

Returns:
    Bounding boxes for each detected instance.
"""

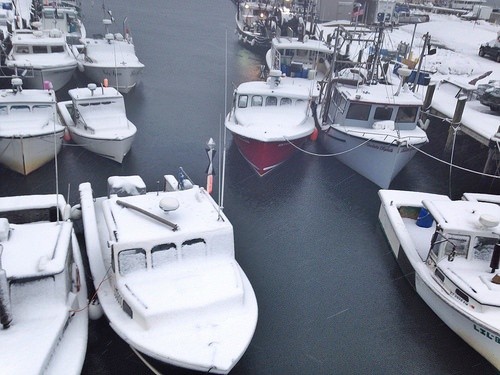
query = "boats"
[234,0,306,48]
[78,166,259,374]
[265,34,429,190]
[0,72,67,176]
[0,0,23,58]
[71,4,145,95]
[0,192,92,374]
[6,25,78,92]
[32,0,87,45]
[223,67,317,176]
[56,79,137,164]
[376,184,499,355]
